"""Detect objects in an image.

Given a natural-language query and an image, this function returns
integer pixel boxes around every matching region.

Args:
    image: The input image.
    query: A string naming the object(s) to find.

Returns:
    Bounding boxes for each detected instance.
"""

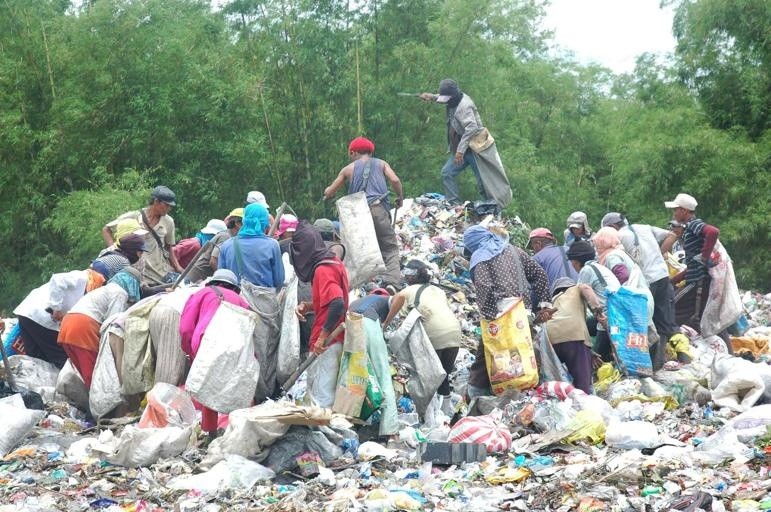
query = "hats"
[246,190,270,209]
[313,218,343,241]
[663,193,699,211]
[602,212,624,226]
[200,217,228,236]
[371,288,390,296]
[551,275,576,297]
[205,268,242,294]
[524,226,555,251]
[435,77,460,106]
[150,185,177,207]
[117,232,150,254]
[667,219,687,227]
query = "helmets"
[224,207,244,224]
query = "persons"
[323,138,403,293]
[418,79,513,213]
[10,187,745,449]
